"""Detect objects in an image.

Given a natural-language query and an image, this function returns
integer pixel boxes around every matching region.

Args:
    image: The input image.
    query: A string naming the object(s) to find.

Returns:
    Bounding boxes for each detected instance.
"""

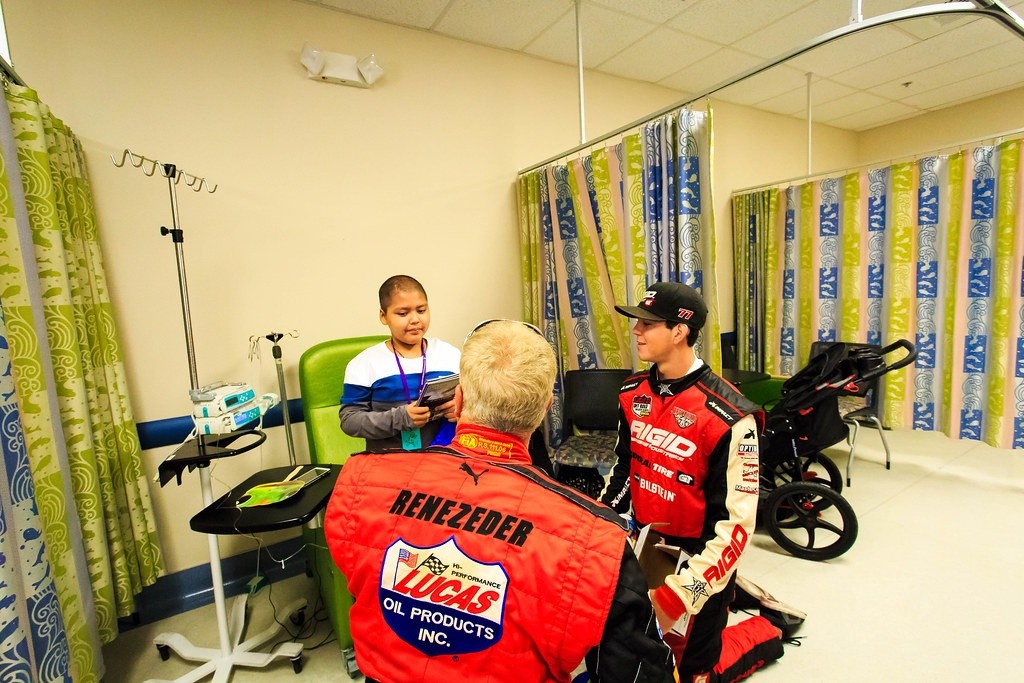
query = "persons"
[338,274,461,451]
[596,281,807,683]
[323,318,685,683]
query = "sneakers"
[760,597,807,647]
[729,573,779,618]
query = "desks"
[723,369,772,386]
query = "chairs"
[807,341,891,489]
[553,368,634,504]
[298,334,392,649]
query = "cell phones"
[293,466,330,489]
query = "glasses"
[464,319,546,344]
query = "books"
[414,373,459,422]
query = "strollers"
[756,339,919,560]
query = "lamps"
[299,41,385,90]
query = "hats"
[614,282,708,329]
